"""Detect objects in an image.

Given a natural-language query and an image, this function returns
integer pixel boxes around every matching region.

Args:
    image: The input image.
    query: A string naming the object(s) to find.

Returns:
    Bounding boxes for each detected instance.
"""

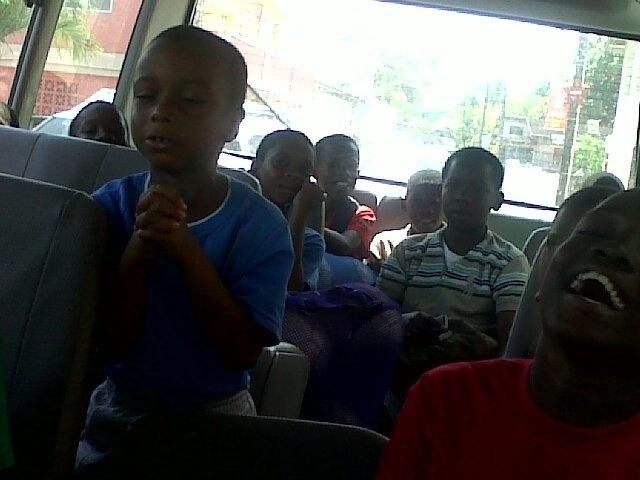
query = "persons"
[310,131,383,291]
[69,25,294,479]
[535,171,627,292]
[365,166,452,281]
[69,100,132,148]
[375,187,640,477]
[374,142,531,393]
[0,101,19,129]
[245,127,405,431]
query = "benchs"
[1,123,412,480]
